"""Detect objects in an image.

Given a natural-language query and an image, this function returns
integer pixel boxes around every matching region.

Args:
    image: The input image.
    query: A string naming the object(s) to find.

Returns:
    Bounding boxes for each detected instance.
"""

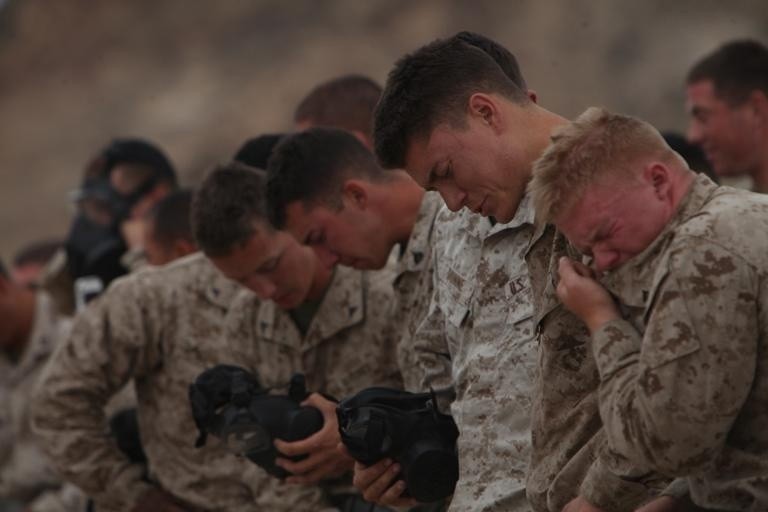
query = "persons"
[3,23,767,511]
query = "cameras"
[334,387,459,502]
[214,393,340,480]
